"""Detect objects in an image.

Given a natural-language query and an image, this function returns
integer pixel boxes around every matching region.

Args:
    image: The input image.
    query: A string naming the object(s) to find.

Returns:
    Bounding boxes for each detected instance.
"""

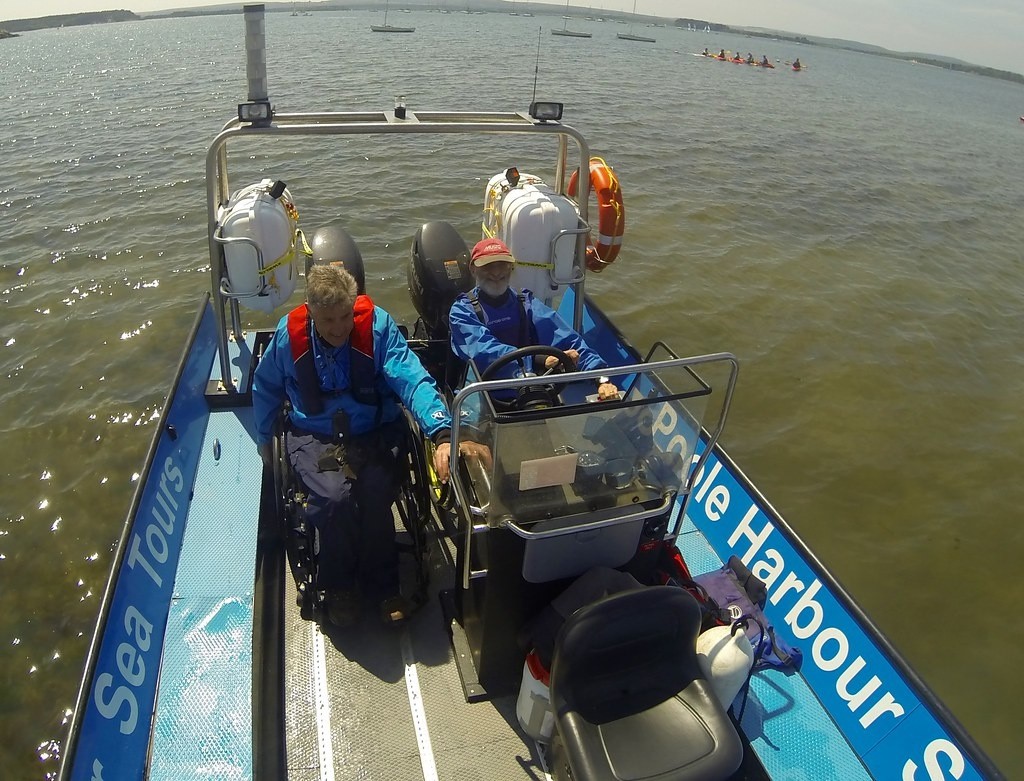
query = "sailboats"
[550,0,593,38]
[371,1,417,31]
[616,0,657,43]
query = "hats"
[469,239,515,268]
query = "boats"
[700,52,775,68]
[792,59,801,71]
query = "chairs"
[536,584,743,781]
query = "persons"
[459,364,493,471]
[251,269,455,622]
[702,48,708,55]
[719,48,725,57]
[747,52,754,62]
[760,55,768,64]
[734,52,741,60]
[793,58,801,68]
[448,238,620,437]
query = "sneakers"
[324,588,355,627]
[381,592,411,625]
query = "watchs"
[596,376,611,384]
